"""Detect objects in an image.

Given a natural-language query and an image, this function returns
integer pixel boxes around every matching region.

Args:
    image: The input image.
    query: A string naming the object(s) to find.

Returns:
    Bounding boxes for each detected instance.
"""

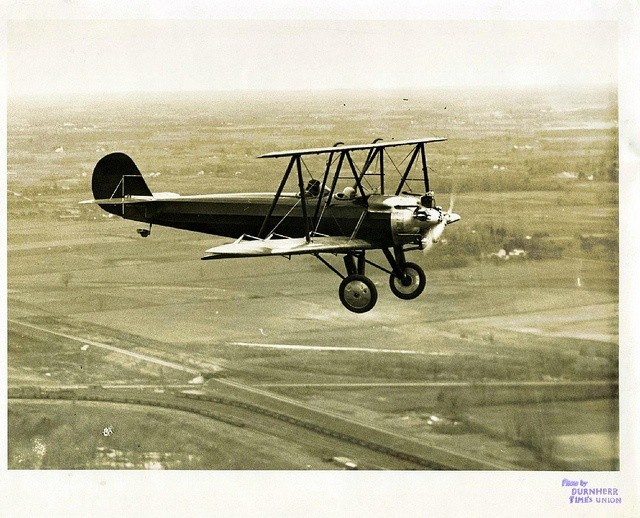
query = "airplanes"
[78,137,461,313]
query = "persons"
[306,179,320,196]
[342,186,357,199]
